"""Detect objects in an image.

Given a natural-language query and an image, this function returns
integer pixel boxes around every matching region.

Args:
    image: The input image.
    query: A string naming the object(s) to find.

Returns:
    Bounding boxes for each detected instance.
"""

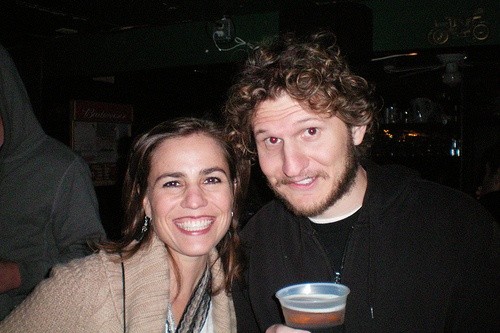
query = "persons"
[1,0,500,332]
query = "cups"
[275,283,350,333]
[379,104,430,140]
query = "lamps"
[432,7,491,45]
